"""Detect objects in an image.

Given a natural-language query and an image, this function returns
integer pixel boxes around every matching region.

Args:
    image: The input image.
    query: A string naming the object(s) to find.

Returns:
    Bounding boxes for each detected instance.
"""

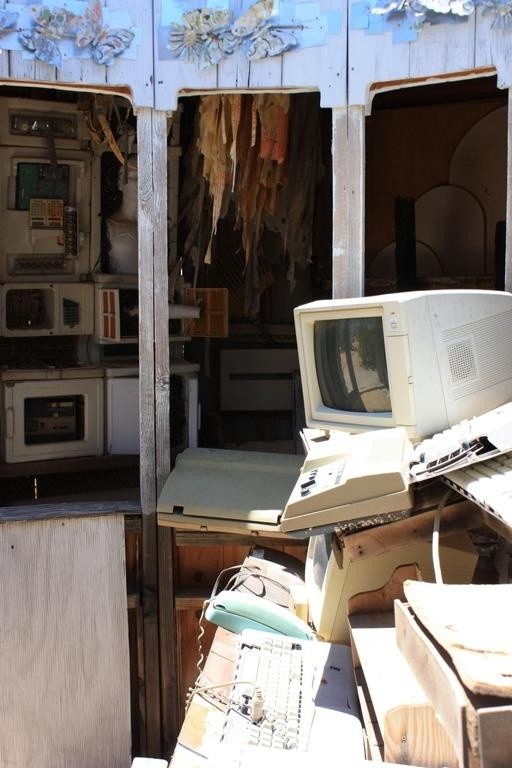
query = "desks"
[167,548,304,768]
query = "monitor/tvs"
[305,527,479,644]
[294,289,511,450]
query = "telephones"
[205,589,316,642]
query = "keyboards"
[441,450,510,523]
[410,400,510,484]
[221,628,365,768]
